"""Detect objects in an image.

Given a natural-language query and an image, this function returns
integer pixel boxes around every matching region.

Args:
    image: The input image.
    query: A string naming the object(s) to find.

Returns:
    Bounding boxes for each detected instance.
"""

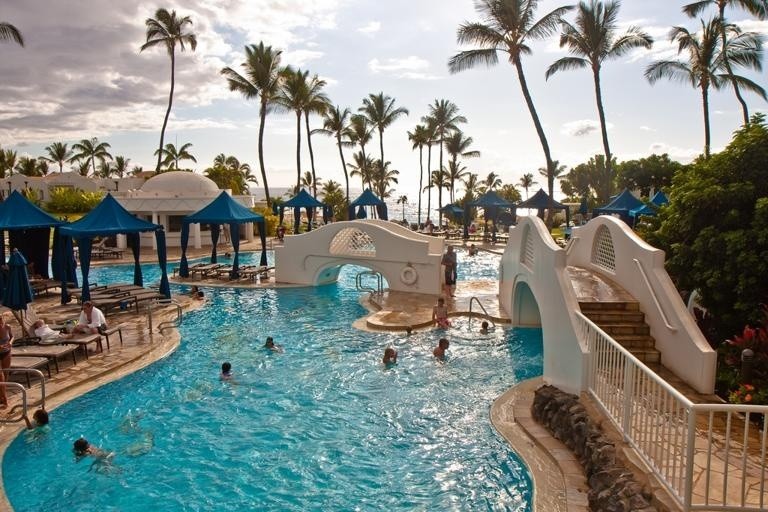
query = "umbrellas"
[2,247,33,341]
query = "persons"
[431,298,449,328]
[468,243,478,257]
[479,321,489,335]
[0,315,13,404]
[432,338,450,363]
[440,245,457,300]
[69,301,108,353]
[21,410,51,438]
[32,320,73,343]
[191,291,209,302]
[261,337,284,354]
[184,285,197,298]
[71,438,105,464]
[398,217,518,245]
[218,362,236,386]
[382,348,398,364]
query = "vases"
[742,403,762,422]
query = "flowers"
[727,382,768,404]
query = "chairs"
[73,243,126,260]
[172,261,275,282]
[0,272,166,388]
[416,226,508,244]
